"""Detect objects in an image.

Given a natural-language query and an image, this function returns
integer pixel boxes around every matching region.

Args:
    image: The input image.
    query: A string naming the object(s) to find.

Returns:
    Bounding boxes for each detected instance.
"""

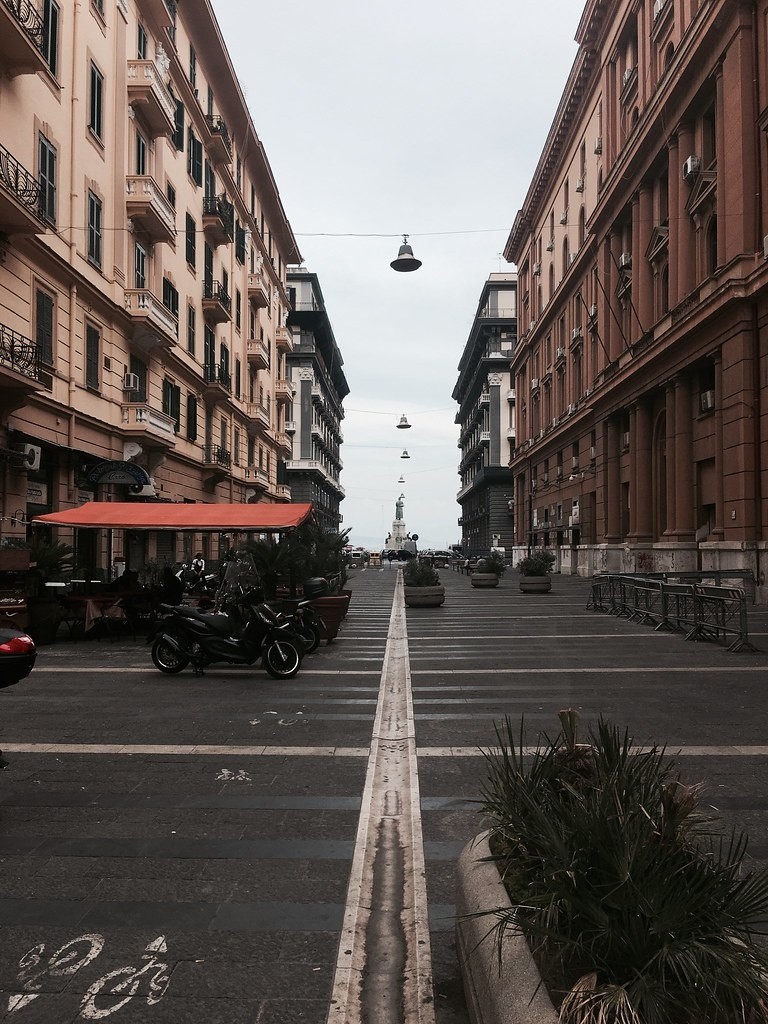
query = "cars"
[342,544,463,569]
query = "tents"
[32,502,318,599]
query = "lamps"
[681,154,699,181]
[396,415,411,428]
[401,449,410,458]
[390,234,422,273]
[623,431,629,447]
[398,475,405,482]
[619,252,630,268]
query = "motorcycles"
[151,549,330,680]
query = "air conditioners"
[623,68,632,85]
[124,373,140,392]
[593,137,601,154]
[699,390,716,412]
[9,442,41,472]
[653,0,666,20]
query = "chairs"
[527,179,596,486]
[52,585,164,643]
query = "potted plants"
[13,533,80,646]
[404,560,447,608]
[471,550,508,587]
[247,521,357,642]
[513,553,552,593]
[454,695,768,1023]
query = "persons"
[465,557,475,575]
[190,553,208,590]
[476,556,486,564]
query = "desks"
[57,594,136,643]
[105,590,163,632]
